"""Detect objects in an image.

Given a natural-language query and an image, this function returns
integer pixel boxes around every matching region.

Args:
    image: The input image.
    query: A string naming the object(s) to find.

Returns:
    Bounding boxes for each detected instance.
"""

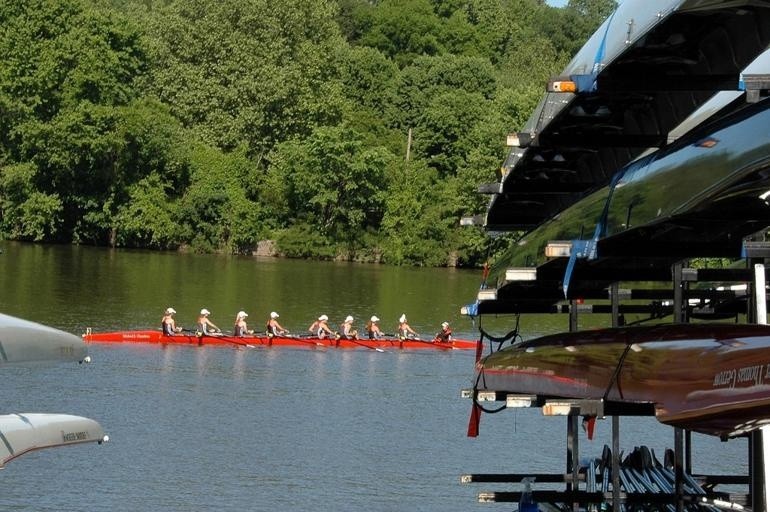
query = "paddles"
[340,337,386,352]
[579,446,724,512]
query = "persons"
[398,314,420,340]
[309,314,360,341]
[160,307,184,337]
[367,315,383,340]
[432,322,452,343]
[196,308,289,342]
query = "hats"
[166,307,280,318]
[318,313,450,326]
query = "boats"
[81,331,486,352]
[482,1,770,234]
[468,315,770,442]
[472,96,770,327]
